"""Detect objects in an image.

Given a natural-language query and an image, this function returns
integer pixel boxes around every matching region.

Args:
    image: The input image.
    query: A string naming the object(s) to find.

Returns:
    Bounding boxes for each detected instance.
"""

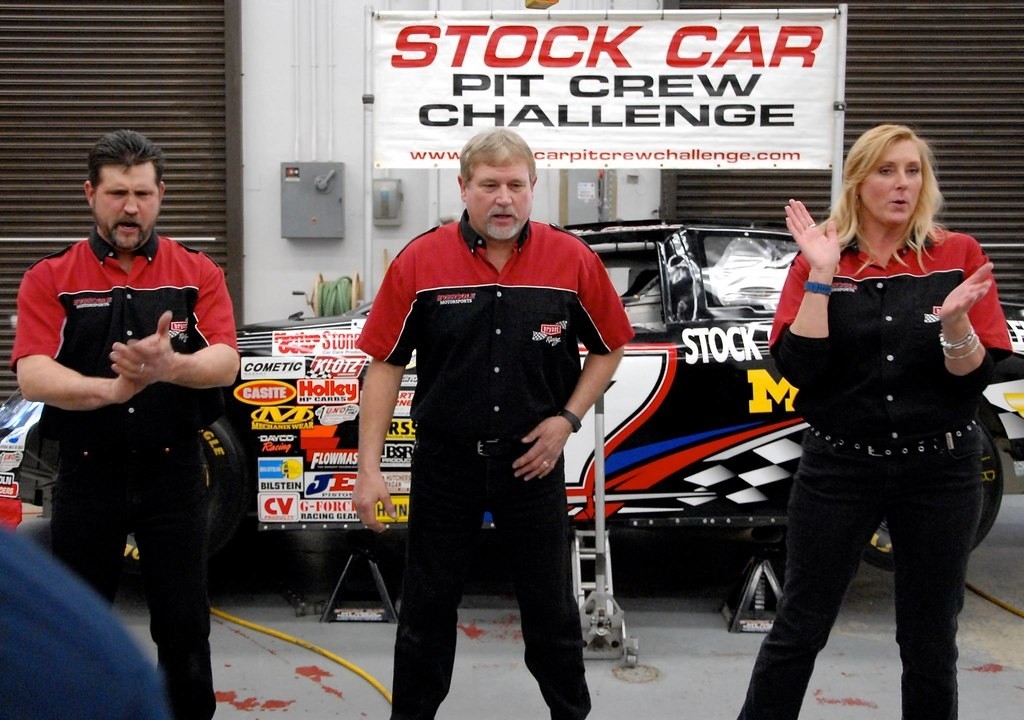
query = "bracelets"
[943,334,979,359]
[940,326,974,350]
[803,281,832,295]
[558,409,582,433]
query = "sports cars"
[0,214,1023,572]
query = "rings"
[139,364,144,373]
[543,461,550,468]
[810,224,816,227]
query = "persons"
[0,525,164,720]
[737,124,1011,720]
[352,129,634,720]
[10,132,240,720]
